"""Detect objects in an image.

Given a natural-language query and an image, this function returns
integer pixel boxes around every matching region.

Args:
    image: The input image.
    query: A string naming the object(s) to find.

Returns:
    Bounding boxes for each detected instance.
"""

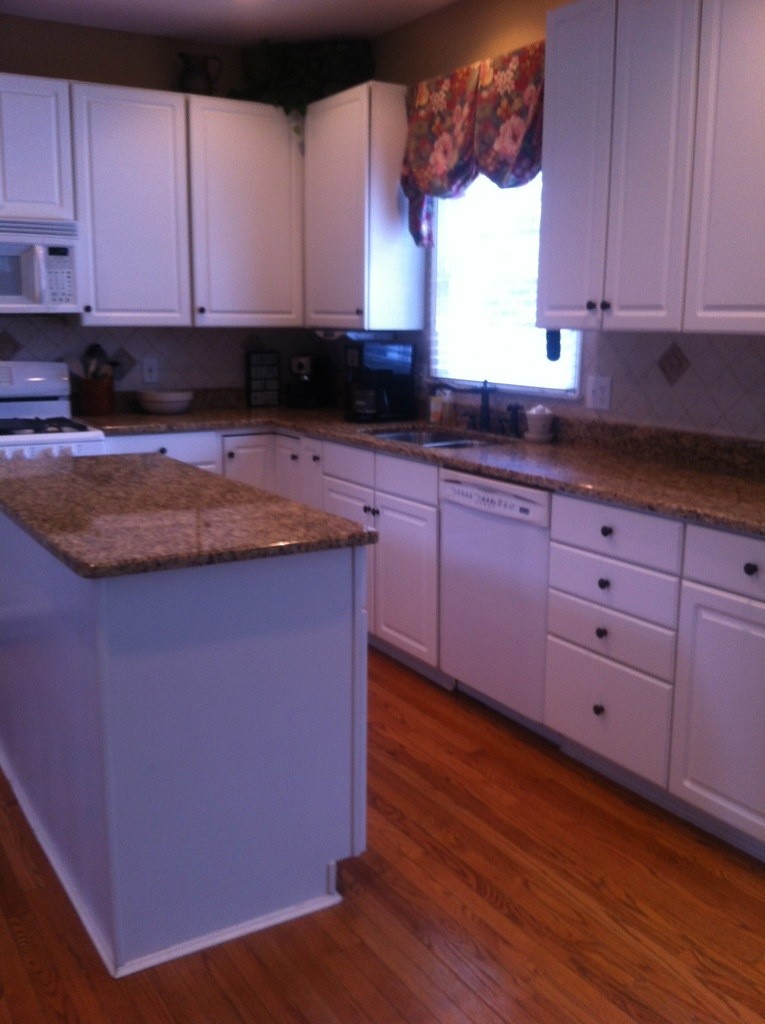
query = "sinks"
[423,439,518,449]
[363,424,469,444]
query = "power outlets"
[587,376,611,412]
[290,355,312,375]
[142,359,161,386]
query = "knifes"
[80,341,105,368]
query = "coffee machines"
[345,342,417,421]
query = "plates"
[524,432,553,443]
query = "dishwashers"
[439,466,550,726]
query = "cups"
[526,411,553,434]
[82,379,112,415]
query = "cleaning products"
[428,376,455,426]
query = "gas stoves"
[0,360,108,460]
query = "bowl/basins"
[140,392,193,413]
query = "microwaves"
[0,218,89,314]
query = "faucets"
[505,403,523,438]
[425,380,498,433]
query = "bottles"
[429,421,456,427]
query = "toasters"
[284,364,337,409]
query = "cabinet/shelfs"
[187,92,304,327]
[223,432,275,486]
[70,80,192,326]
[320,442,437,684]
[535,0,701,333]
[303,80,426,331]
[683,0,765,335]
[0,73,75,220]
[547,488,683,821]
[668,521,765,864]
[106,429,219,479]
[300,435,323,509]
[270,431,303,503]
